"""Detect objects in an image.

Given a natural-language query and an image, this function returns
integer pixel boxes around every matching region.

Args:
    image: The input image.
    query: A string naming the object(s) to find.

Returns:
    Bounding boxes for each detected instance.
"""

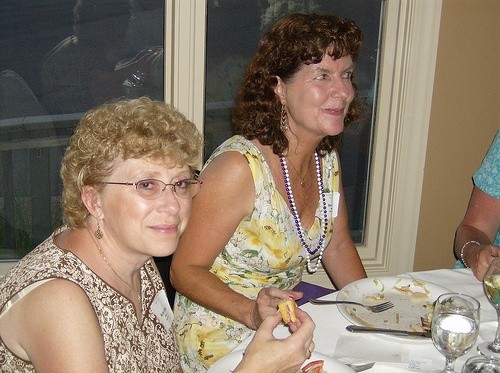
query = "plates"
[205,347,355,373]
[336,275,460,343]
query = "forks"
[345,362,375,372]
[308,298,394,313]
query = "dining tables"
[230,267,500,373]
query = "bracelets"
[461,241,480,268]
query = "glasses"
[85,175,203,200]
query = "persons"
[453,130,500,281]
[169,13,367,373]
[0,96,316,373]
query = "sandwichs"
[278,298,297,324]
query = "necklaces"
[86,227,141,303]
[280,152,328,272]
[286,157,307,188]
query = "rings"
[306,350,311,359]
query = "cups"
[459,355,500,373]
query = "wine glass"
[431,293,481,373]
[478,257,500,359]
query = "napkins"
[291,281,336,306]
